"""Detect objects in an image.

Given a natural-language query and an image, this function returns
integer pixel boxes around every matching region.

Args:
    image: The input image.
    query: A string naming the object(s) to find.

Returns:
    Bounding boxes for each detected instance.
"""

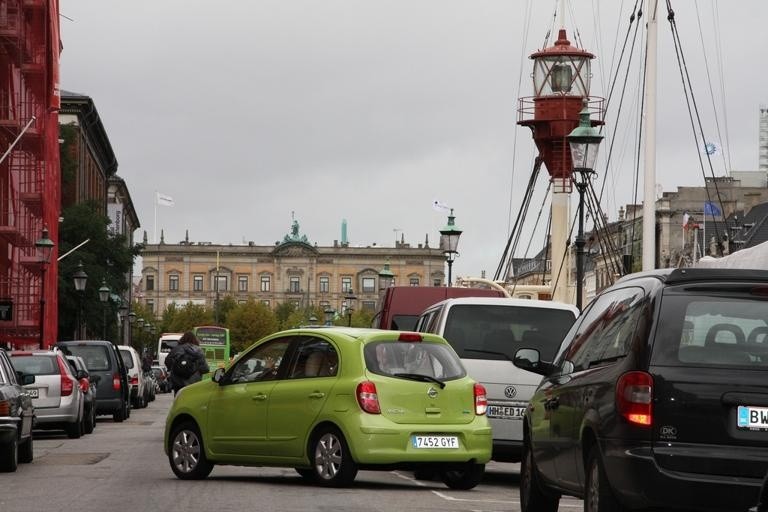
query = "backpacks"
[172,352,199,381]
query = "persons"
[255,355,276,381]
[162,332,212,397]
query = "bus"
[188,325,232,384]
[158,331,185,371]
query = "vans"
[414,296,582,465]
[367,285,508,333]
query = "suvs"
[512,265,768,512]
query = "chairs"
[703,323,767,352]
[454,323,541,345]
[387,367,434,378]
[39,359,51,373]
[93,353,105,367]
[14,359,26,372]
[304,342,324,377]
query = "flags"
[683,213,691,229]
[703,201,722,217]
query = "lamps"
[34,223,56,261]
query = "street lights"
[374,251,397,310]
[441,204,466,286]
[96,276,157,364]
[35,222,55,348]
[73,257,89,341]
[308,285,358,326]
[566,96,604,314]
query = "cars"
[165,326,498,496]
[237,355,266,374]
[0,339,171,476]
